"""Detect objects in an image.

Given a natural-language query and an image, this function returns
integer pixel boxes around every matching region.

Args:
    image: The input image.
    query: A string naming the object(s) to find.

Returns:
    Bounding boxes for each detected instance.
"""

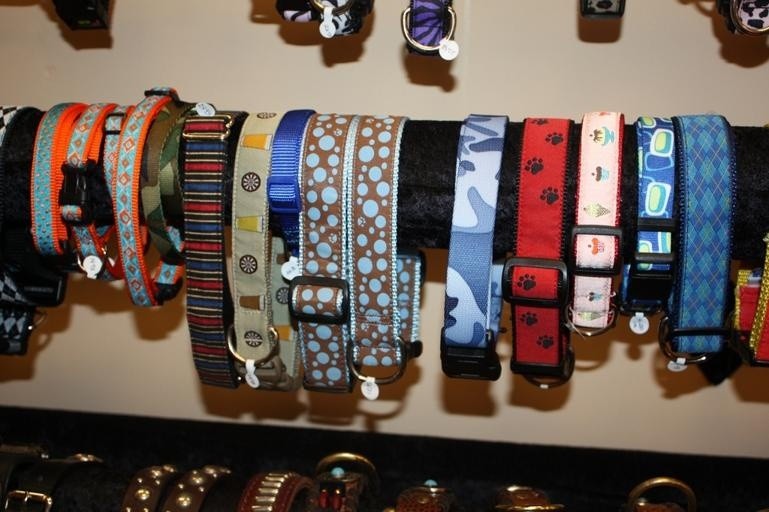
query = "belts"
[565,111,625,336]
[502,118,574,389]
[730,232,769,367]
[51,0,109,30]
[401,0,459,60]
[0,86,424,393]
[0,446,696,512]
[579,0,625,18]
[441,115,509,380]
[275,0,372,36]
[620,115,736,365]
[716,0,769,38]
